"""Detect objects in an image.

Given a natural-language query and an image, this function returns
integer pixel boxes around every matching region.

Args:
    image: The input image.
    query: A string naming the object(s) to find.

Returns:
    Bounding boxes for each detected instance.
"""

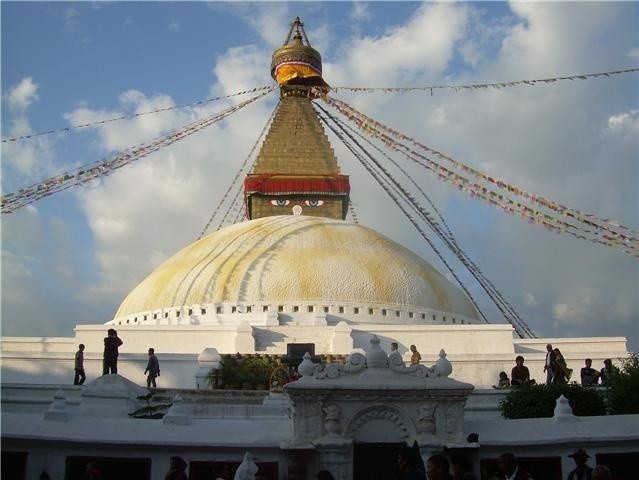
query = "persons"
[72,344,86,385]
[398,448,455,479]
[451,450,480,479]
[390,342,398,352]
[144,348,160,388]
[84,461,106,480]
[235,452,263,479]
[316,469,334,479]
[566,448,611,480]
[409,345,420,365]
[166,456,188,478]
[492,343,617,389]
[498,452,533,480]
[102,329,124,375]
[481,459,498,479]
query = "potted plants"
[205,353,286,389]
